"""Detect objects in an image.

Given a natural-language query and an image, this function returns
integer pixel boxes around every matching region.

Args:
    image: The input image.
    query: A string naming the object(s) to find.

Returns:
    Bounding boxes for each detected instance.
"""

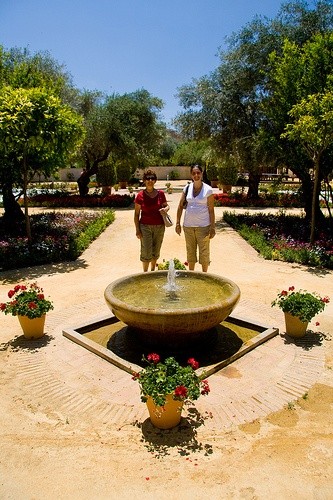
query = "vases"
[284,312,308,337]
[144,394,184,429]
[17,312,46,339]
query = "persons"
[175,164,216,272]
[134,169,170,273]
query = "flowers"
[132,353,211,411]
[0,281,54,320]
[271,286,330,326]
[155,258,189,270]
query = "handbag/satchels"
[180,185,189,209]
[160,209,173,227]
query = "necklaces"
[145,189,154,194]
[194,188,199,193]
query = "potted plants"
[167,188,174,194]
[128,186,133,193]
[114,185,120,191]
[165,183,171,188]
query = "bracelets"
[176,223,179,225]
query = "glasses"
[145,177,155,181]
